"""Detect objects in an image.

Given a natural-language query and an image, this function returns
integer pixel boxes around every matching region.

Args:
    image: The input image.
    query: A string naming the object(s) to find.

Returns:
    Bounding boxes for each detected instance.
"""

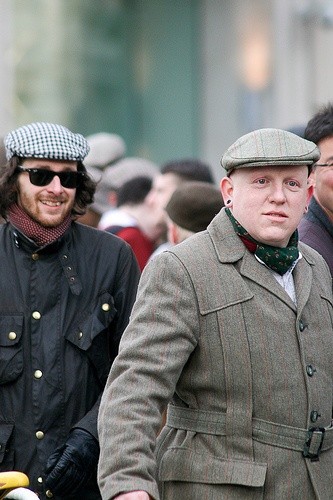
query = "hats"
[167,183,225,232]
[5,122,90,161]
[220,129,321,177]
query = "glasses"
[17,166,85,189]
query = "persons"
[97,128,333,500]
[76,133,226,272]
[0,122,141,500]
[298,104,333,276]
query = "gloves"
[45,428,100,494]
